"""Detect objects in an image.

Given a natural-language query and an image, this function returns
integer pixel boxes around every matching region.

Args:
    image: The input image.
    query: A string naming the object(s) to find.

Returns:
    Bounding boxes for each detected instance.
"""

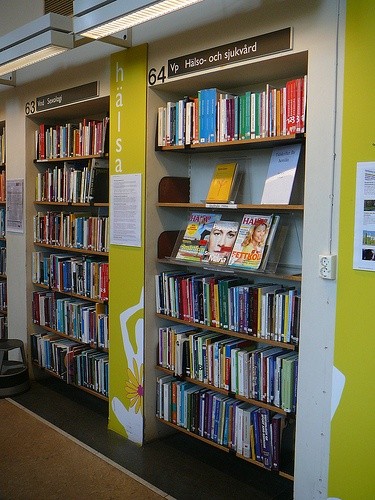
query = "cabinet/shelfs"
[152,49,309,482]
[30,95,110,402]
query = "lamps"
[0,13,74,75]
[73,0,204,40]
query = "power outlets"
[317,253,336,280]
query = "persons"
[232,220,268,274]
[184,213,216,256]
[208,229,237,263]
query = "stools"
[0,338,30,398]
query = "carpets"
[0,398,177,500]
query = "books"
[156,76,306,472]
[0,128,7,339]
[31,117,108,398]
